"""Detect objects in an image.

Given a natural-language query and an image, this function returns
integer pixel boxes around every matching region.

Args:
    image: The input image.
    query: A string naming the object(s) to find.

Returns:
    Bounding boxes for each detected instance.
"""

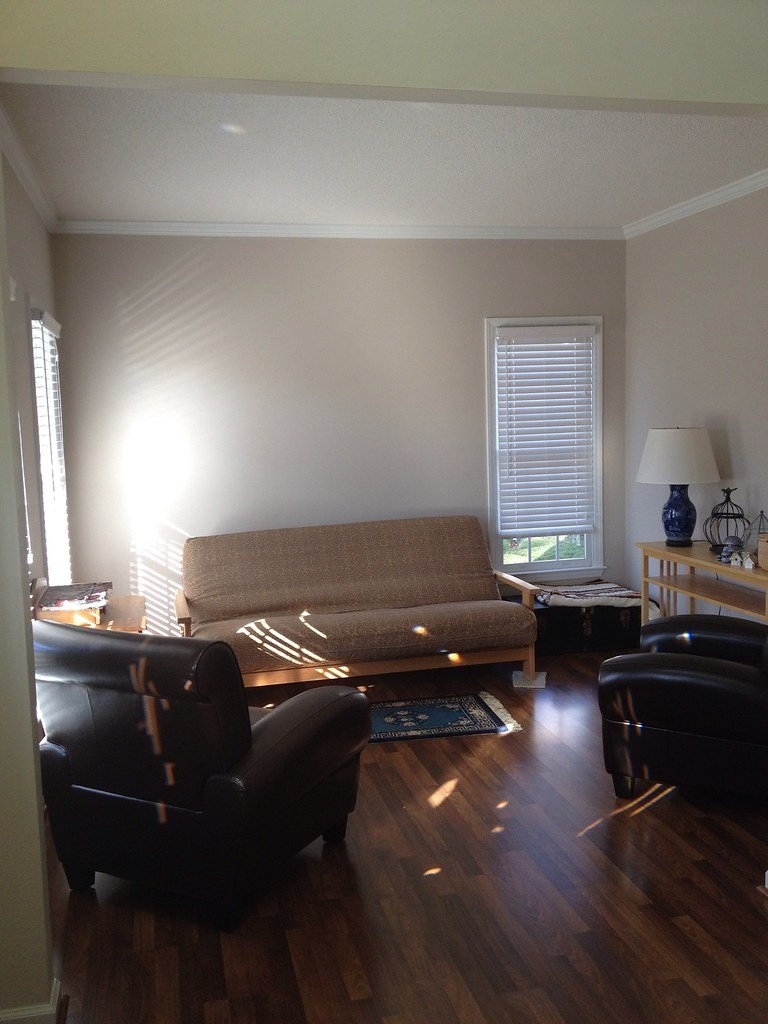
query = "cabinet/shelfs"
[34,604,106,625]
[503,592,640,658]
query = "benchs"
[174,515,542,688]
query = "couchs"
[31,617,368,935]
[597,615,767,800]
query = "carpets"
[369,690,523,743]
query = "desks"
[78,594,146,633]
[634,541,768,626]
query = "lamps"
[635,426,721,548]
